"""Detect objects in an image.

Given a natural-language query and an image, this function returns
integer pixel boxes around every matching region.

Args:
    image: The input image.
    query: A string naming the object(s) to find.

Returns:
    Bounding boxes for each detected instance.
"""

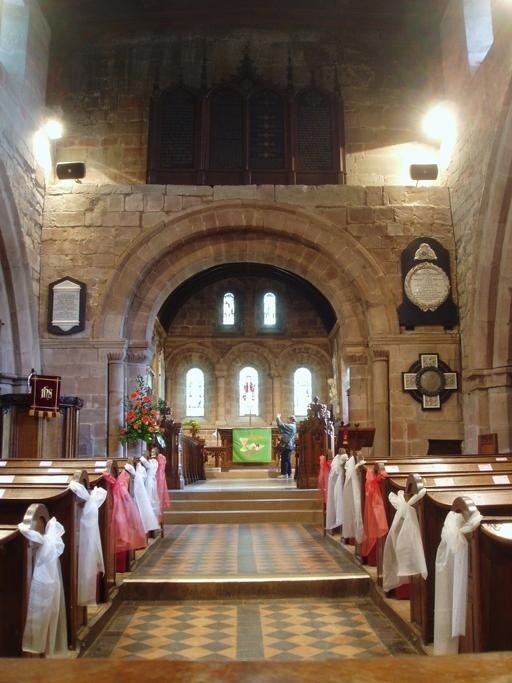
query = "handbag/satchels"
[276,441,288,454]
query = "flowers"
[116,373,168,456]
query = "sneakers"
[278,474,292,479]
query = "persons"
[275,412,297,479]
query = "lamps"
[56,161,86,184]
[409,163,439,189]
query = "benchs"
[164,422,206,490]
[0,455,165,660]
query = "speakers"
[409,163,438,181]
[56,161,87,179]
